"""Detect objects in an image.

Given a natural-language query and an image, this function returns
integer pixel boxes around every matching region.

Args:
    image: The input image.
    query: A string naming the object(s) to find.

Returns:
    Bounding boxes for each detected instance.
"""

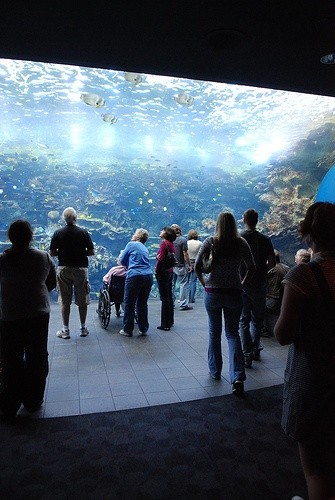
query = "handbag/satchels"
[159,242,178,269]
[200,238,216,274]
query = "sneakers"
[119,329,133,337]
[56,329,70,339]
[138,330,148,336]
[79,326,89,336]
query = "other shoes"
[245,361,252,368]
[180,306,193,310]
[232,378,244,392]
[27,400,43,412]
[209,372,221,380]
[157,326,170,330]
[252,355,260,361]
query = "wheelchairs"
[94,274,139,330]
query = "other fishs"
[1,59,335,299]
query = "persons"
[167,224,193,310]
[184,230,203,302]
[119,228,154,337]
[96,250,132,314]
[0,219,57,423]
[154,226,176,331]
[231,210,276,369]
[292,249,311,266]
[194,212,256,392]
[260,249,290,337]
[270,202,335,500]
[49,207,95,339]
